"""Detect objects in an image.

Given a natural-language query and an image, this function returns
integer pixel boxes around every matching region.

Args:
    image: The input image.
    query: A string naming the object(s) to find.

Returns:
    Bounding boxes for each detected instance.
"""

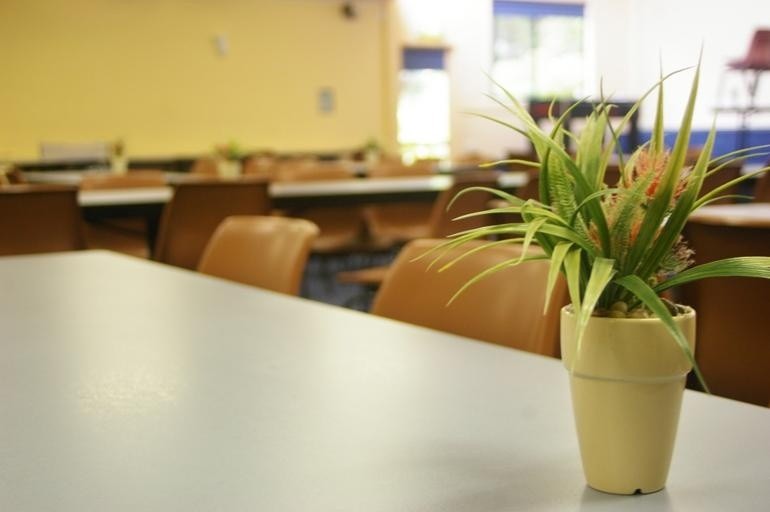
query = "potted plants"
[402,39,770,495]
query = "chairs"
[367,233,565,359]
[193,211,319,299]
[149,177,271,273]
[336,172,503,309]
[0,181,83,256]
[673,211,768,407]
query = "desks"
[0,161,769,211]
[2,243,770,510]
